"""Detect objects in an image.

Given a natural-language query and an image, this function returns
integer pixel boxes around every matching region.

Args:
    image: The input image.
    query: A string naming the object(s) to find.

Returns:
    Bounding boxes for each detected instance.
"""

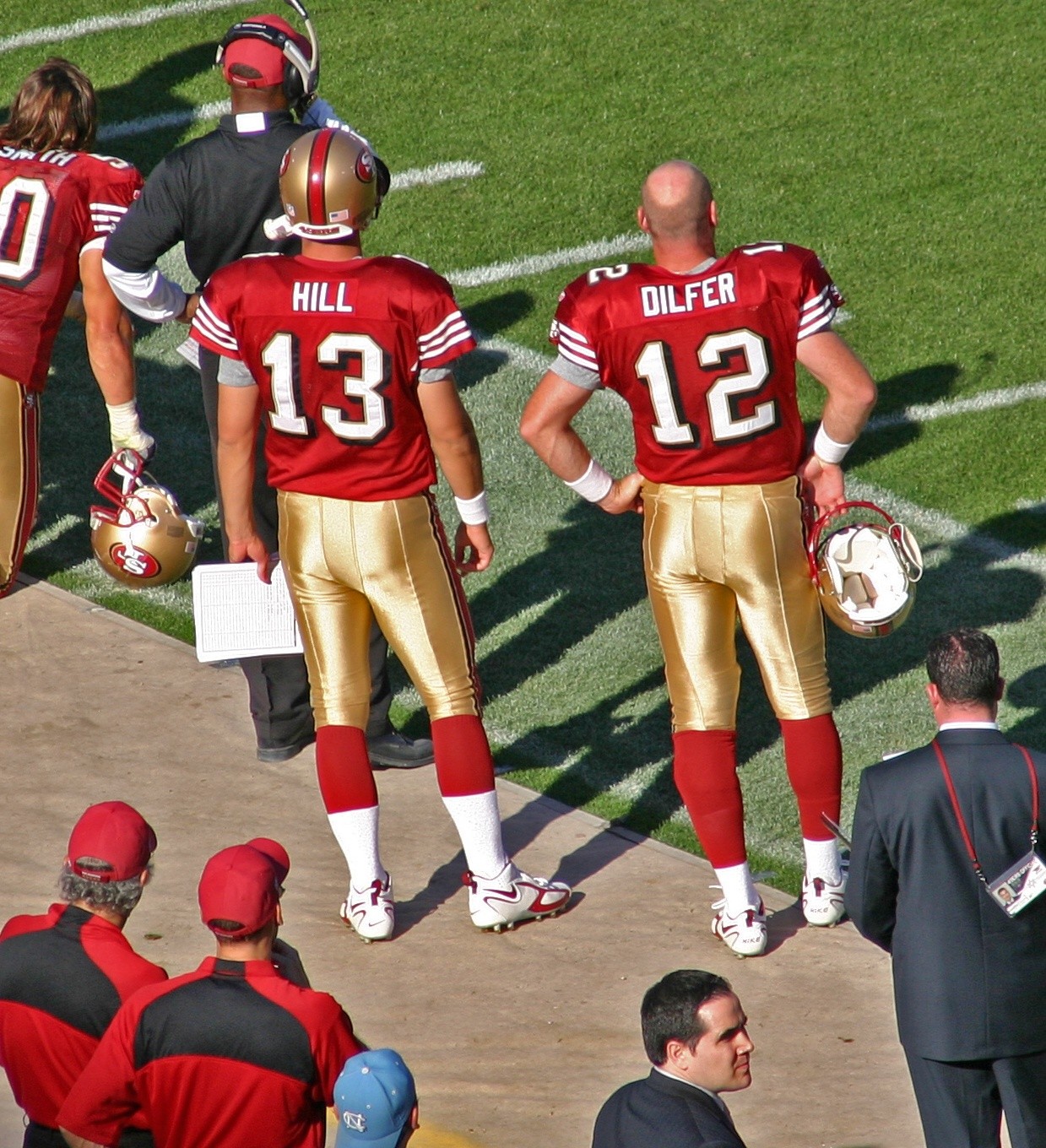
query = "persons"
[335,1049,420,1148]
[997,888,1019,908]
[189,124,571,944]
[54,837,363,1148]
[844,633,1046,1148]
[0,54,157,603]
[592,969,754,1148]
[102,0,433,767]
[0,800,169,1148]
[520,159,877,960]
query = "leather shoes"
[366,729,434,767]
[256,731,317,761]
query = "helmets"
[807,501,924,639]
[90,446,203,588]
[280,127,390,241]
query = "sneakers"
[711,894,768,956]
[803,869,848,927]
[340,870,395,940]
[463,852,571,933]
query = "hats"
[197,837,290,935]
[68,801,158,882]
[333,1049,415,1148]
[222,15,312,88]
